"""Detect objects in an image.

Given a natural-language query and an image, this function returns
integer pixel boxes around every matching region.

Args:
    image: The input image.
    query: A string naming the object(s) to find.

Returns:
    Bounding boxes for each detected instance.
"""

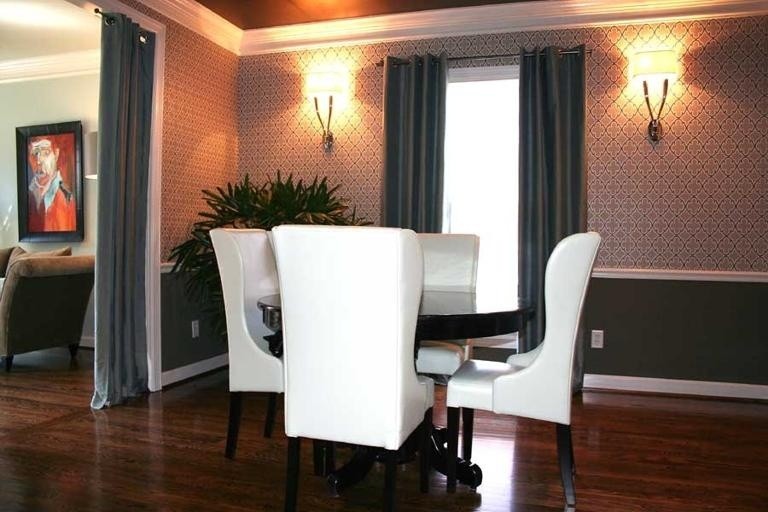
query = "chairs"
[273,223,434,512]
[446,231,602,506]
[0,254,95,371]
[209,227,336,477]
[412,230,480,460]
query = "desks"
[256,289,536,496]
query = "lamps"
[633,50,681,143]
[303,70,348,151]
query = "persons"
[24,136,76,231]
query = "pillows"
[5,246,72,278]
[0,246,15,278]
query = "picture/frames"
[15,120,84,243]
